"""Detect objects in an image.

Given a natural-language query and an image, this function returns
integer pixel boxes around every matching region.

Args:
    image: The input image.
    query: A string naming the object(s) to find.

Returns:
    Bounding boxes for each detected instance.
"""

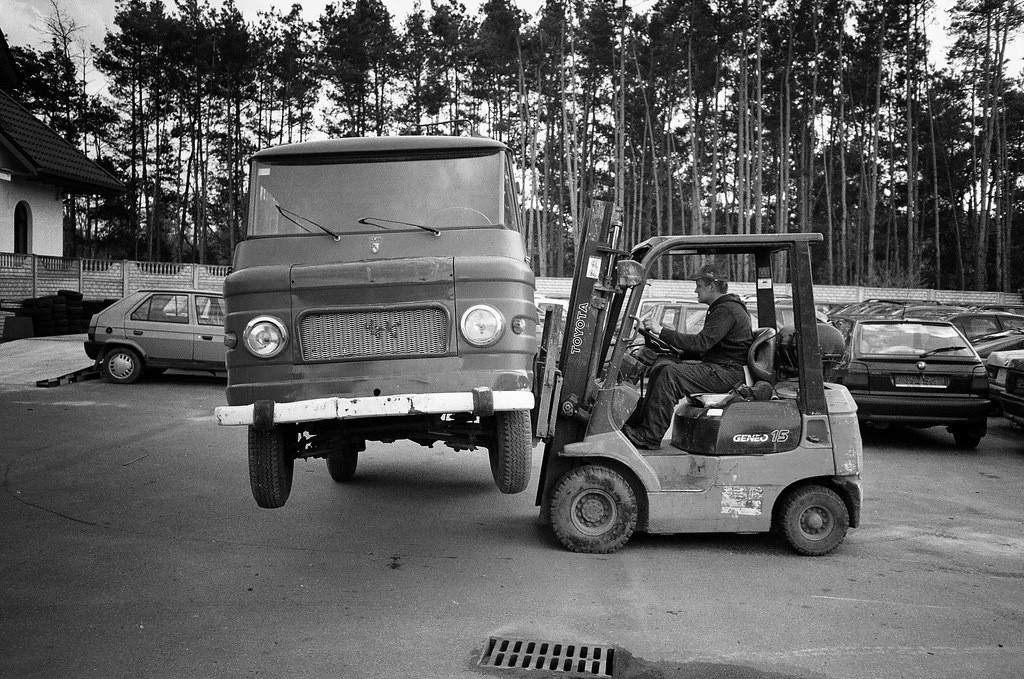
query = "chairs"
[687,328,776,407]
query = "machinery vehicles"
[538,204,864,558]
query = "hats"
[691,265,730,282]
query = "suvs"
[737,294,829,338]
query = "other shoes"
[624,424,660,451]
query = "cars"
[986,349,1024,438]
[820,296,906,335]
[933,310,1024,359]
[631,297,710,337]
[830,317,989,450]
[83,289,228,384]
[875,304,968,330]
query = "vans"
[216,135,539,509]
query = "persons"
[625,264,758,451]
